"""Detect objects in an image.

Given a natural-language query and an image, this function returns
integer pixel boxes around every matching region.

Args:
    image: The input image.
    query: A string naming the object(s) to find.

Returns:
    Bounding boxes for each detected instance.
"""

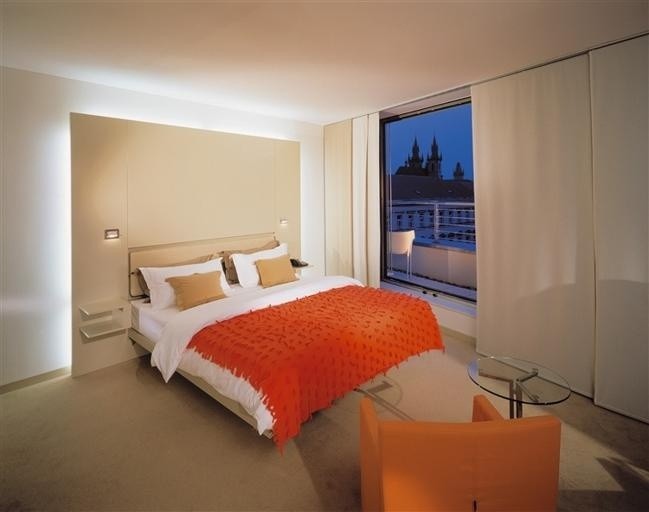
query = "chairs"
[387,229,417,279]
[358,392,561,512]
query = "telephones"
[290,258,308,267]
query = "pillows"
[135,238,301,312]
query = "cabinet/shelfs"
[78,298,129,340]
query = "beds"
[128,234,447,454]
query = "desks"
[467,354,572,419]
